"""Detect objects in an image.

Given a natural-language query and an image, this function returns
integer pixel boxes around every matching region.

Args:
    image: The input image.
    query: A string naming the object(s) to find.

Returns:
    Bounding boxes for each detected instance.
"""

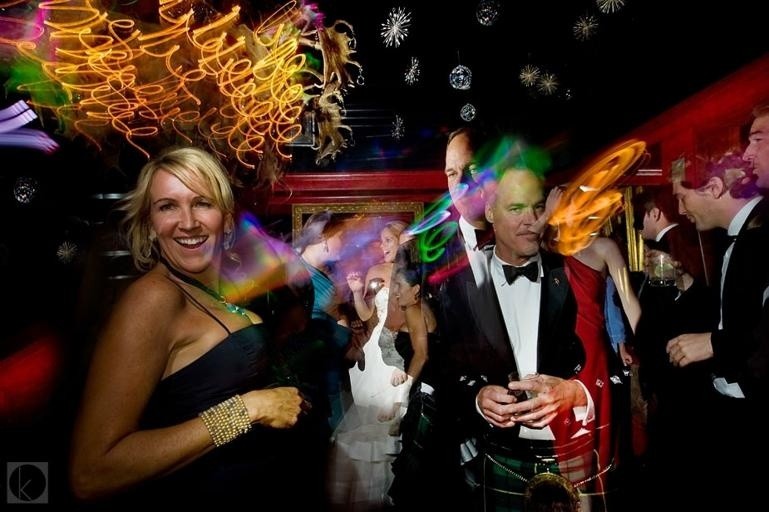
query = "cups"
[507,370,541,425]
[647,252,681,289]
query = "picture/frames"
[292,202,424,361]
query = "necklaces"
[162,259,249,321]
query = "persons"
[67,143,323,509]
[663,139,768,403]
[744,97,768,187]
[426,124,523,291]
[434,164,587,512]
[62,182,769,511]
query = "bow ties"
[500,259,539,285]
[473,228,496,250]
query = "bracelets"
[196,394,253,449]
